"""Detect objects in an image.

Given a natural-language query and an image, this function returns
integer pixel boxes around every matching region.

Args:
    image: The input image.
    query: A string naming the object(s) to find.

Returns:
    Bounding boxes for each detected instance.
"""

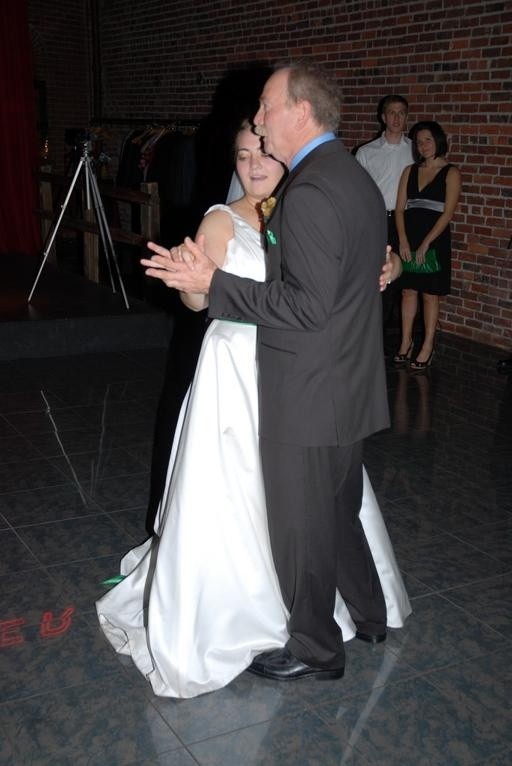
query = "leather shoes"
[244,646,344,680]
[355,630,387,644]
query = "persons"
[388,361,433,533]
[93,112,411,699]
[355,95,416,323]
[139,56,390,683]
[395,120,461,370]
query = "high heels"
[393,339,414,363]
[409,344,435,369]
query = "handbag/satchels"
[400,249,440,273]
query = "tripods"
[24,156,134,315]
